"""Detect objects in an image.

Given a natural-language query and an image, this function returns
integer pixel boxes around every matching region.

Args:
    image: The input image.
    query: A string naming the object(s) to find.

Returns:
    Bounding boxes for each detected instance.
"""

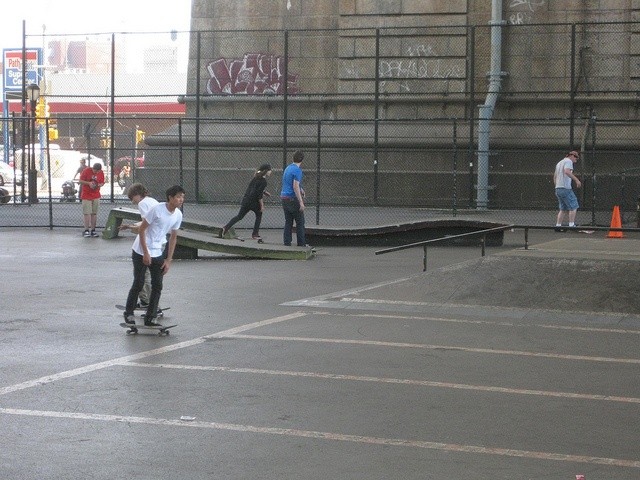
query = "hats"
[566,151,579,157]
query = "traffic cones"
[606,206,625,238]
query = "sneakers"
[144,319,163,327]
[123,311,137,326]
[252,234,261,241]
[83,231,91,238]
[91,231,99,237]
[219,227,225,239]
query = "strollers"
[61,178,79,202]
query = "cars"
[0,161,28,186]
[80,153,104,173]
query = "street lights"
[27,84,40,205]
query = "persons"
[74,159,87,203]
[121,161,131,186]
[218,164,272,239]
[127,183,160,309]
[553,151,582,231]
[280,152,316,252]
[123,185,185,326]
[78,163,105,237]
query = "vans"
[112,156,145,187]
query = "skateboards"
[220,235,264,244]
[120,323,177,336]
[116,304,170,316]
